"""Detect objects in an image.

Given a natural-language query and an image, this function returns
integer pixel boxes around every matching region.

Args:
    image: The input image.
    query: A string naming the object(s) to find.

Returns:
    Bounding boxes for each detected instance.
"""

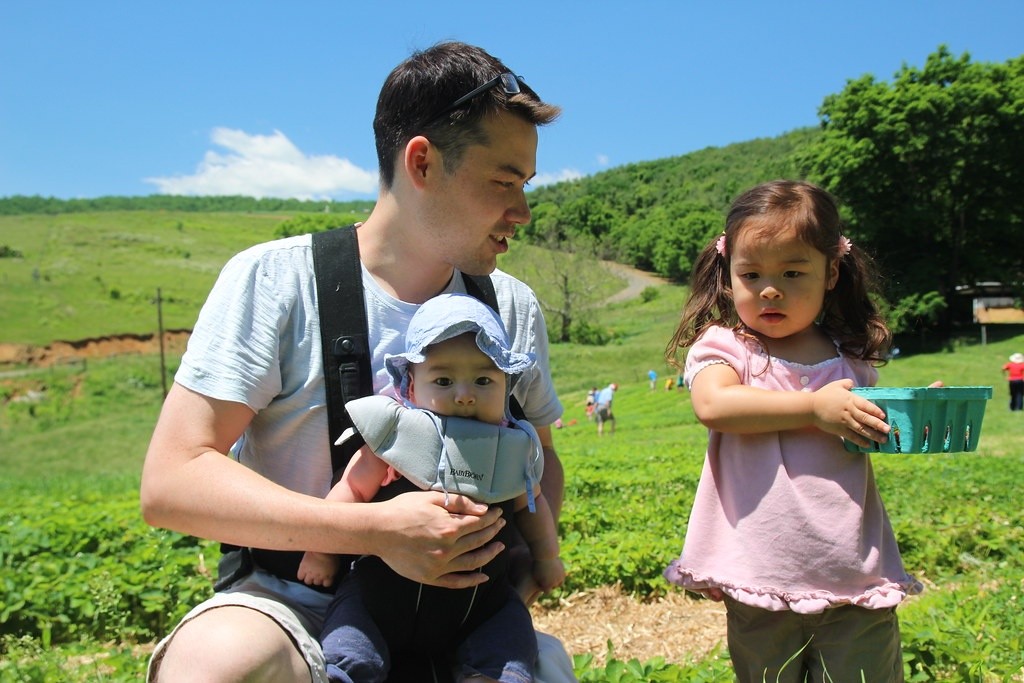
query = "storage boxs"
[841,385,994,456]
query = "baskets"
[846,384,993,453]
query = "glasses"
[411,74,526,140]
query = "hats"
[382,294,532,395]
[1009,353,1024,363]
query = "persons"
[585,383,619,435]
[1002,353,1024,411]
[663,179,944,683]
[648,368,684,393]
[141,42,577,683]
